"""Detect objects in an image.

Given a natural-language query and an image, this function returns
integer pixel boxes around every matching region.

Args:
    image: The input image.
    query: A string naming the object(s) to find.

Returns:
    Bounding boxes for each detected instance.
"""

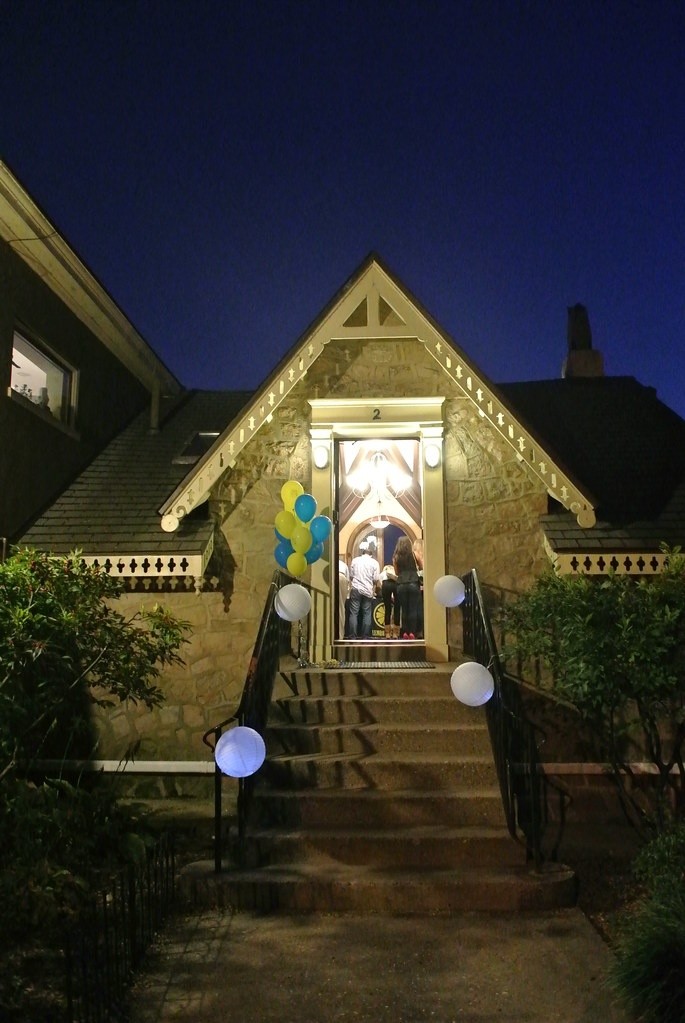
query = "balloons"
[295,494,317,523]
[274,543,294,569]
[434,575,465,607]
[274,583,311,621]
[286,553,307,576]
[305,541,324,564]
[450,662,495,706]
[310,516,333,541]
[215,727,265,777]
[281,481,304,510]
[291,527,312,553]
[274,510,310,544]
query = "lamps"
[345,453,413,529]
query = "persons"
[337,561,351,640]
[393,536,424,639]
[381,564,400,639]
[347,550,380,640]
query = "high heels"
[403,633,409,639]
[409,633,415,639]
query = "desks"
[344,598,401,638]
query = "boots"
[385,624,391,639]
[392,624,400,639]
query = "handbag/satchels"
[386,568,398,581]
[417,570,423,585]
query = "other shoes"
[363,635,373,639]
[348,636,356,640]
